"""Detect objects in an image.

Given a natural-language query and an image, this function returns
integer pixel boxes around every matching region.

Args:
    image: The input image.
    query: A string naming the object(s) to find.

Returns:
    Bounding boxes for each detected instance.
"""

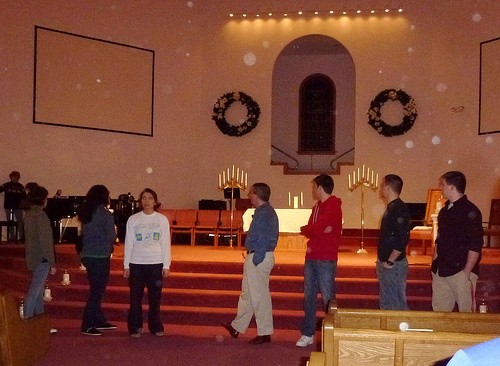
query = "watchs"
[387,260,394,266]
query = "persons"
[221,183,279,343]
[431,171,483,312]
[376,174,411,311]
[22,186,55,317]
[79,185,118,335]
[124,188,171,338]
[296,175,343,347]
[0,171,26,240]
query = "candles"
[218,165,248,187]
[348,164,378,188]
[288,191,304,209]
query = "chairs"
[404,188,449,256]
[156,209,242,250]
[482,198,500,249]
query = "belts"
[247,250,271,254]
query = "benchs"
[306,299,500,366]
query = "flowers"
[211,92,261,137]
[361,88,417,136]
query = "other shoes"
[81,326,101,335]
[156,332,164,336]
[95,321,117,329]
[130,332,140,337]
[221,318,239,338]
[248,336,271,344]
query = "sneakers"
[295,334,314,346]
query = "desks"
[243,208,346,253]
[0,221,25,245]
[59,218,82,244]
